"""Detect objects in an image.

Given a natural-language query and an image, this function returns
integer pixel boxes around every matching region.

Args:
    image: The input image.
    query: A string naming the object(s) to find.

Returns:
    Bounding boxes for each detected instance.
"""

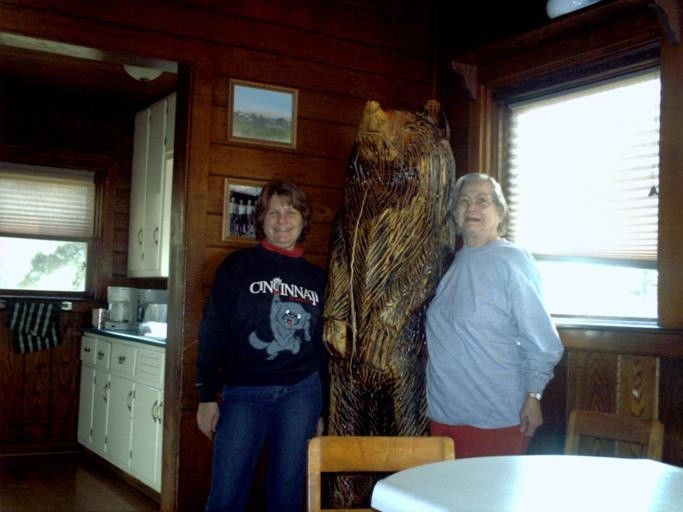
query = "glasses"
[457,196,494,207]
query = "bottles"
[137,305,144,321]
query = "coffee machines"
[103,286,138,330]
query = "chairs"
[305,436,455,512]
[561,408,665,461]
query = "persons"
[195,176,327,511]
[424,173,564,456]
[229,192,257,235]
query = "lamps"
[123,64,164,82]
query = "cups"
[96,308,106,329]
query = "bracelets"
[529,392,544,402]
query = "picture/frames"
[226,78,299,151]
[221,177,272,245]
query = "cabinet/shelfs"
[76,332,166,495]
[125,93,177,279]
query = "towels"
[6,301,65,355]
